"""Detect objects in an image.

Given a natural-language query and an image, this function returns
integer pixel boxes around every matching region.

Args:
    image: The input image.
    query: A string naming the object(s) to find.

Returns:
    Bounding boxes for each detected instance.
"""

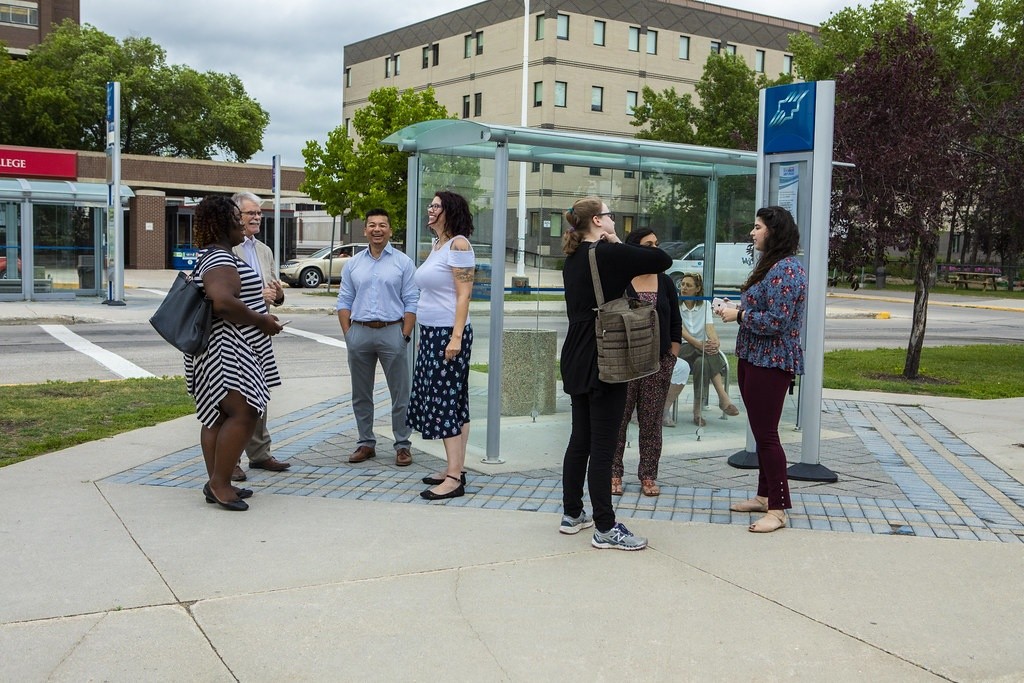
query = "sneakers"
[559,508,594,535]
[591,523,648,550]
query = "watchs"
[403,334,411,342]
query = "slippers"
[641,480,660,496]
[611,478,623,495]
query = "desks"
[952,271,1001,291]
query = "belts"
[353,319,403,329]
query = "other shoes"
[719,402,739,416]
[422,471,466,486]
[420,475,466,500]
[693,416,706,427]
[663,415,676,427]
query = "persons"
[406,191,475,499]
[337,208,421,466]
[662,356,690,427]
[712,207,808,533]
[560,198,673,551]
[182,196,283,511]
[678,275,739,425]
[231,192,290,480]
[612,227,683,495]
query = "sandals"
[729,497,768,512]
[748,512,787,533]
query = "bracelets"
[737,309,744,324]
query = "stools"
[671,348,730,423]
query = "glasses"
[426,203,442,211]
[239,210,262,217]
[592,213,615,222]
[685,271,702,281]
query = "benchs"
[952,280,1000,291]
[0,279,51,294]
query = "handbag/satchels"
[149,247,237,355]
[589,238,661,383]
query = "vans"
[662,241,755,294]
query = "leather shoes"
[206,488,253,503]
[249,456,291,471]
[231,466,247,481]
[203,480,249,511]
[348,445,376,462]
[396,448,412,466]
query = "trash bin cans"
[76,255,95,289]
[471,264,491,301]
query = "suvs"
[279,242,369,289]
